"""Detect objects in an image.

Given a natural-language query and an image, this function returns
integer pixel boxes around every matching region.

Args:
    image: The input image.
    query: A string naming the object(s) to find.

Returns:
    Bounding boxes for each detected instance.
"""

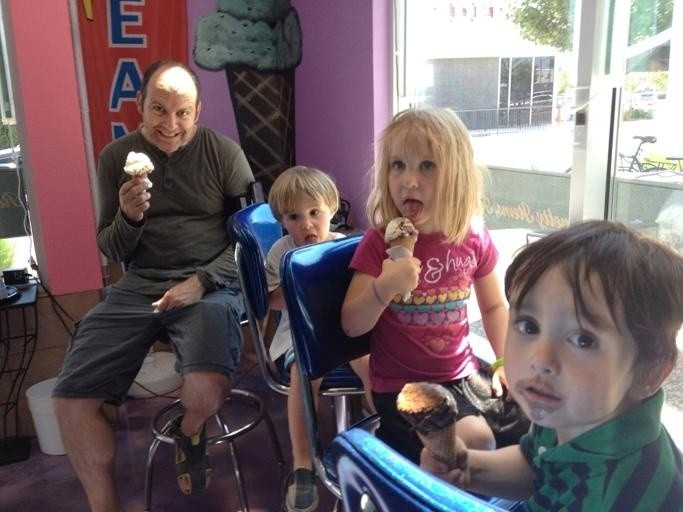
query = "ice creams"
[384,219,422,258]
[125,151,156,177]
[395,380,460,465]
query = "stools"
[139,306,284,511]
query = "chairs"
[280,232,386,510]
[226,194,374,444]
[322,408,541,511]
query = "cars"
[508,89,551,109]
[623,84,664,111]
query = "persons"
[419,220,683,512]
[52,60,256,512]
[265,165,377,512]
[341,108,531,465]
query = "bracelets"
[372,280,389,307]
[491,358,505,371]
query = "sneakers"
[285,469,319,512]
[171,413,212,496]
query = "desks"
[0,281,46,470]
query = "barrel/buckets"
[25,376,67,456]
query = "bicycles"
[564,132,682,182]
